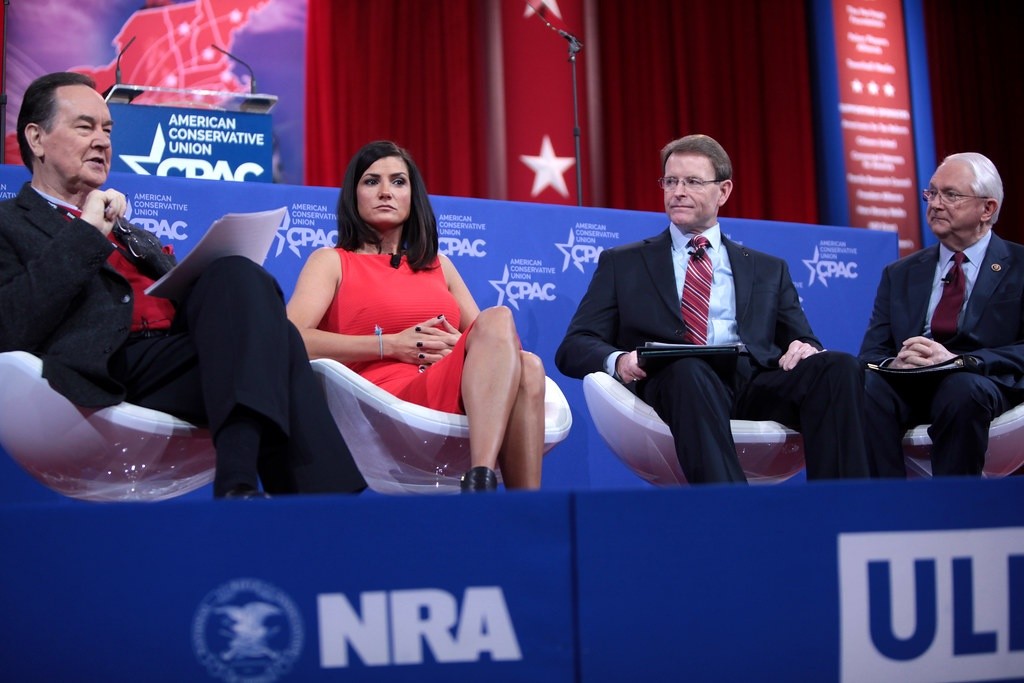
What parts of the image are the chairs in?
[309,358,571,493]
[903,402,1024,478]
[584,371,809,484]
[0,346,217,504]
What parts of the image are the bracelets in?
[375,325,385,361]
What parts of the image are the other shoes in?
[460,466,497,494]
[222,489,271,501]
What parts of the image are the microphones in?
[390,254,401,269]
[210,43,257,94]
[694,248,705,259]
[116,36,136,84]
[945,273,953,281]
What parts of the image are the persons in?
[555,136,861,485]
[856,150,1024,475]
[287,140,544,492]
[0,73,373,498]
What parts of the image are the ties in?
[931,252,966,344]
[680,234,713,346]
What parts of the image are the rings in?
[633,378,637,382]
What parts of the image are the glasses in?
[657,176,736,192]
[117,193,142,258]
[922,189,990,204]
